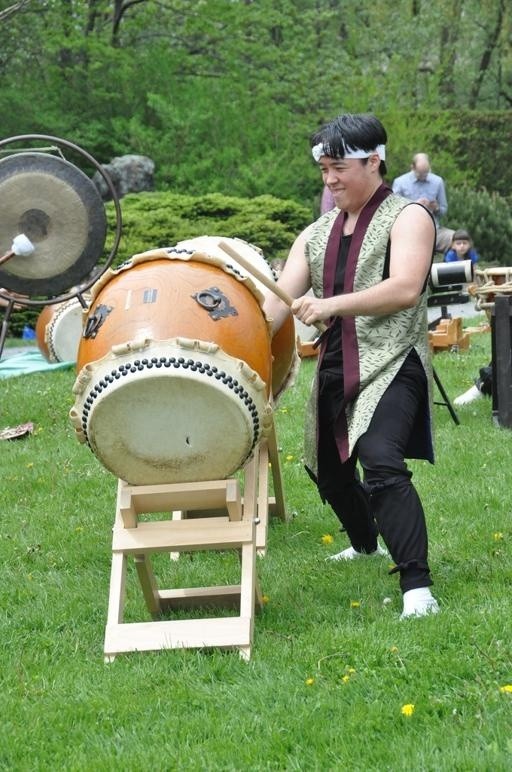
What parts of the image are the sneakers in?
[398,599,441,622]
[453,383,484,405]
[323,543,389,561]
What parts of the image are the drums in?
[175,235,301,408]
[36,294,95,365]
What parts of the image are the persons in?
[454,358,492,405]
[320,184,336,215]
[392,153,456,252]
[264,113,438,621]
[445,228,479,265]
[0,287,28,310]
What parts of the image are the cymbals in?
[0,152,108,297]
[69,247,275,485]
